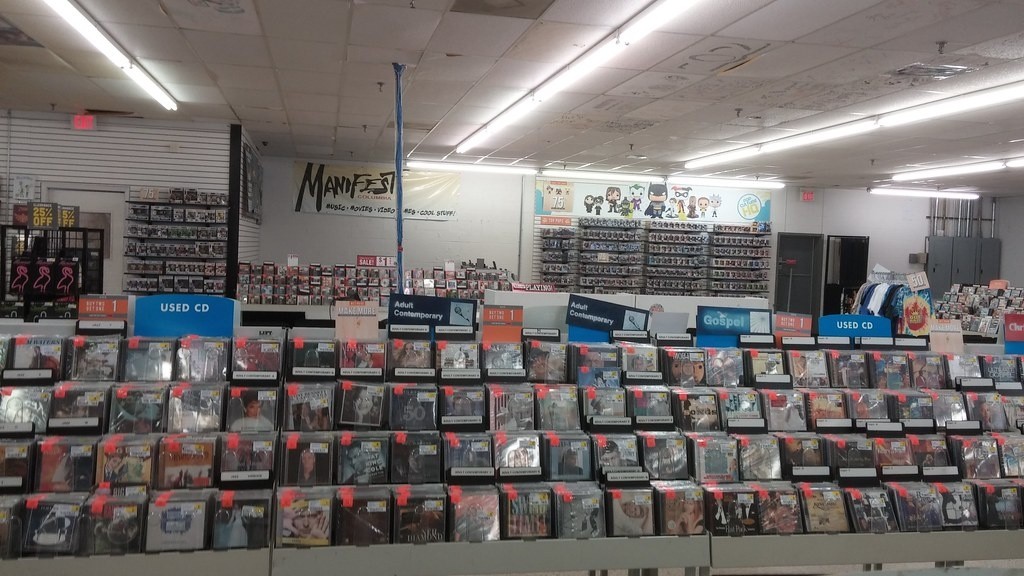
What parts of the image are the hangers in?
[864,272,909,285]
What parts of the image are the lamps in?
[405,159,787,189]
[456,0,703,154]
[866,188,982,200]
[44,0,178,112]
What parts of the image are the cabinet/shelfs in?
[122,200,229,295]
[644,228,703,291]
[576,226,644,288]
[539,236,576,287]
[707,232,772,294]
[928,236,1002,299]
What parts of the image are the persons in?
[4,336,1024,554]
